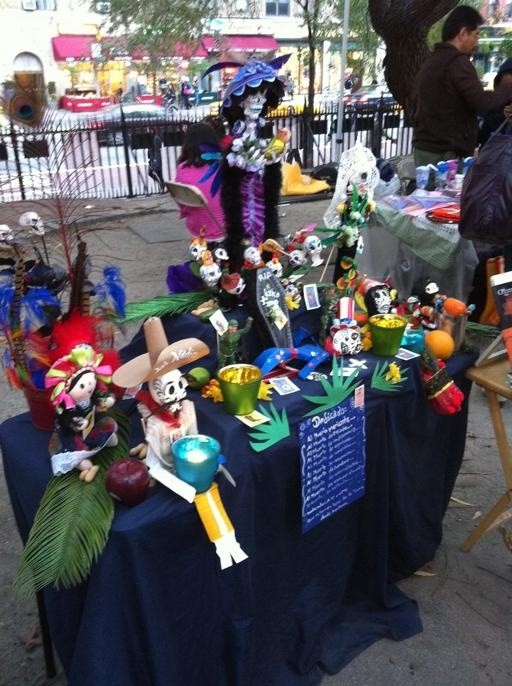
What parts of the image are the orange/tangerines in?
[187,367,210,388]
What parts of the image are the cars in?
[335,84,398,114]
[78,101,325,147]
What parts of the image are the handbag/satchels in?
[458,116,511,244]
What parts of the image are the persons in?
[462,56,512,324]
[286,72,294,100]
[161,75,201,117]
[370,75,378,85]
[344,78,353,89]
[404,4,488,166]
[147,126,165,194]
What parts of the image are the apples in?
[105,460,149,503]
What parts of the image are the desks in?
[1,302,479,684]
[359,173,492,321]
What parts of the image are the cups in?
[369,313,407,357]
[216,363,263,415]
[434,303,467,353]
[173,435,222,493]
